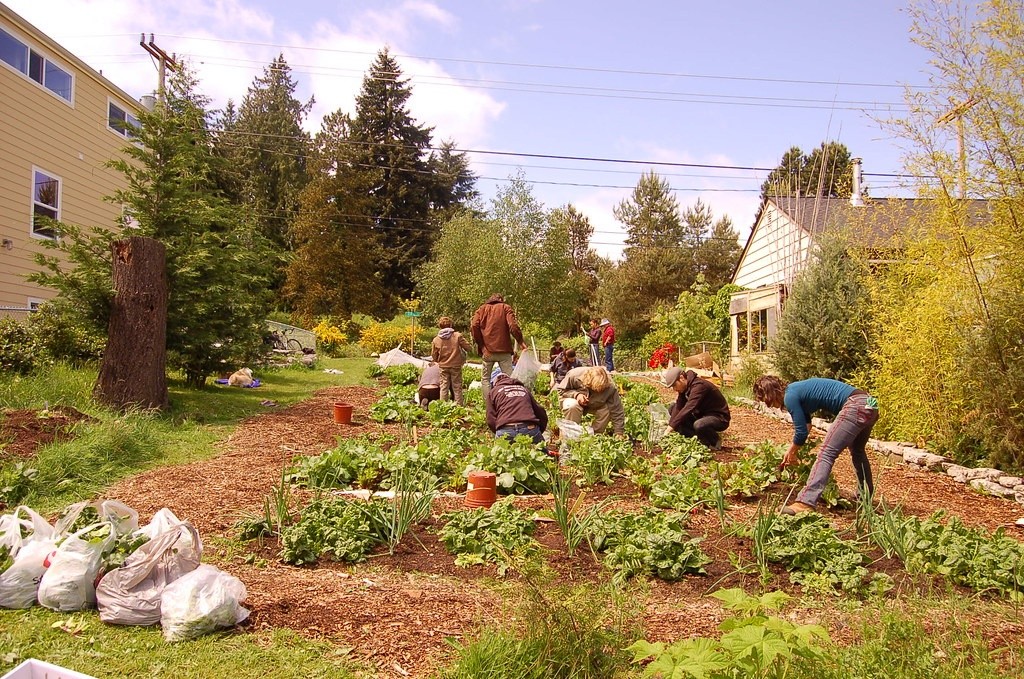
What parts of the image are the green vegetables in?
[0,506,152,574]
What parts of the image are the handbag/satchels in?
[509,349,541,387]
[0,500,251,642]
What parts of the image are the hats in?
[666,367,683,388]
[599,318,610,326]
[493,373,509,387]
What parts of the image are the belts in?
[502,422,531,429]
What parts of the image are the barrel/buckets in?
[334,402,353,424]
[464,470,496,509]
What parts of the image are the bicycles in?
[273,328,302,351]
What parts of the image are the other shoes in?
[420,398,428,411]
[778,502,815,516]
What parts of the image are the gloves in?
[865,397,879,411]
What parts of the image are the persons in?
[472,294,524,399]
[663,368,731,451]
[550,348,582,387]
[558,366,625,442]
[580,319,602,366]
[490,352,518,388]
[428,316,476,408]
[417,364,453,411]
[486,372,548,453]
[549,342,564,364]
[599,318,615,373]
[752,375,879,516]
